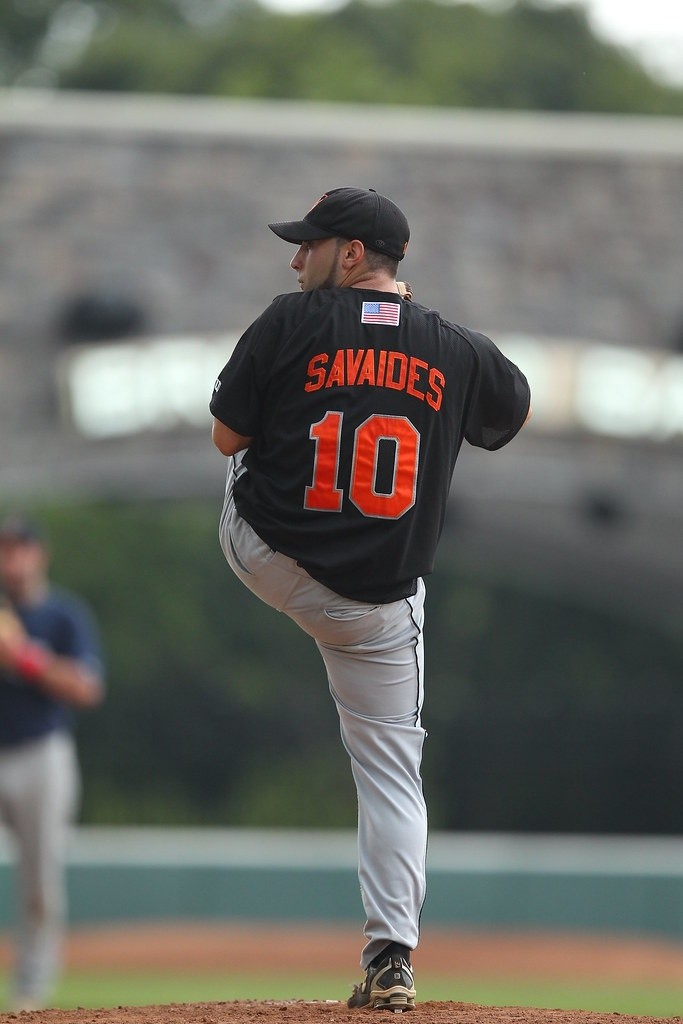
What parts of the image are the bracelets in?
[17,641,49,681]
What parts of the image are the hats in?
[268,188,409,261]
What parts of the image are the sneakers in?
[343,953,417,1012]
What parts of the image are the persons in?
[206,186,532,1009]
[0,519,106,1004]
